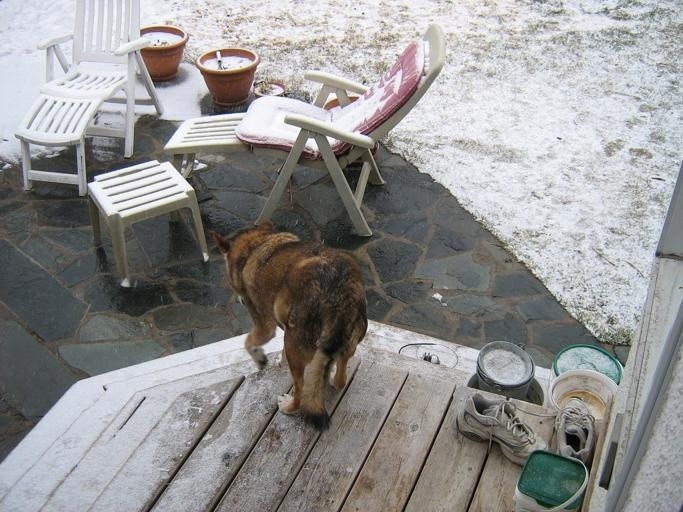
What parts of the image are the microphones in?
[82,160,212,290]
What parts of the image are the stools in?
[82,160,212,290]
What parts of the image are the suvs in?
[550,344,623,383]
[511,449,591,511]
[475,341,536,398]
[550,370,619,432]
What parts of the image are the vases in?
[140,26,190,81]
[198,46,260,106]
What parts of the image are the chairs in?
[17,1,162,197]
[554,397,594,465]
[163,22,445,237]
[455,392,556,467]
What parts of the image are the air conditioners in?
[207,219,369,432]
